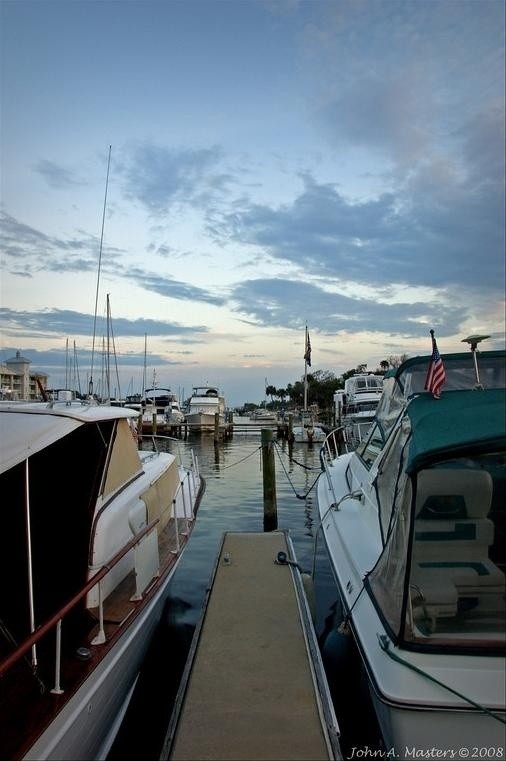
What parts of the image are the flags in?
[424,338,448,400]
[304,333,312,367]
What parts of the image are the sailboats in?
[0,292,506,440]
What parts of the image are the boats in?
[311,332,505,760]
[2,400,207,760]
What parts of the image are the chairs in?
[416,468,506,617]
